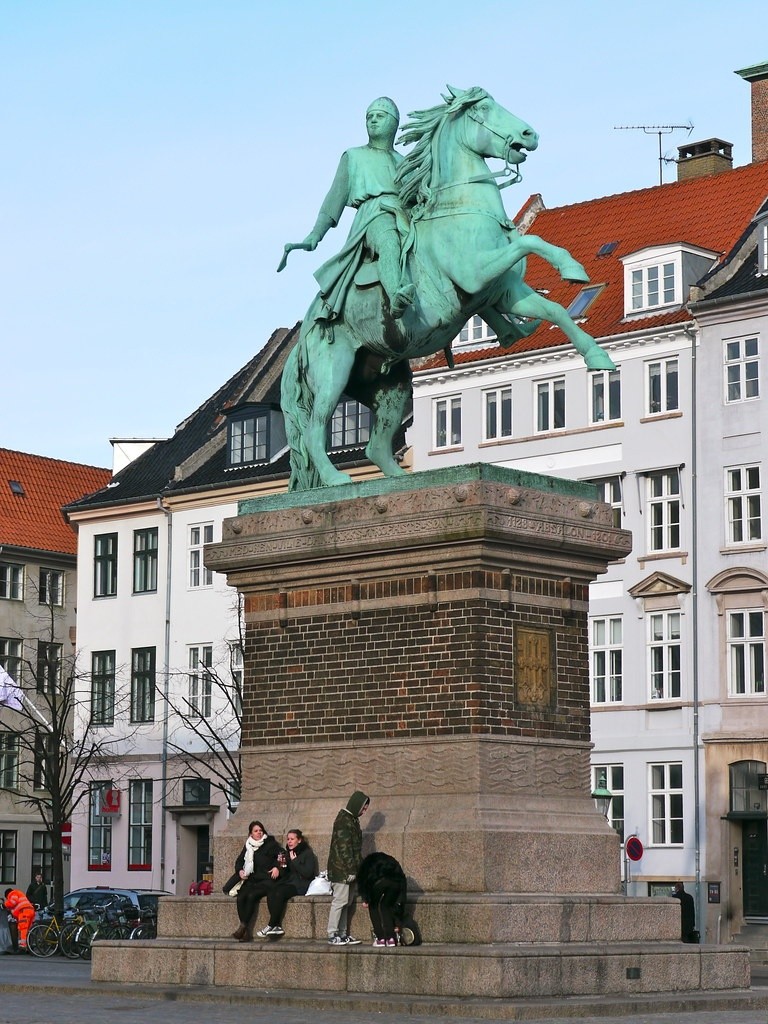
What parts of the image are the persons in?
[302,96,426,319]
[232,820,287,942]
[671,882,696,942]
[1,888,35,955]
[25,873,48,909]
[257,828,320,938]
[356,851,408,948]
[326,790,370,946]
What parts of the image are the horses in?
[278,82,619,494]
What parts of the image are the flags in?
[0,666,24,711]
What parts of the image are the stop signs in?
[61,823,72,846]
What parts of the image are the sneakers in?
[373,938,395,947]
[343,936,362,945]
[257,925,285,937]
[233,924,252,941]
[328,936,347,945]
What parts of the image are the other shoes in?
[17,948,30,954]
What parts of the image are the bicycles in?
[25,903,157,960]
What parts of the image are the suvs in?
[33,886,176,921]
